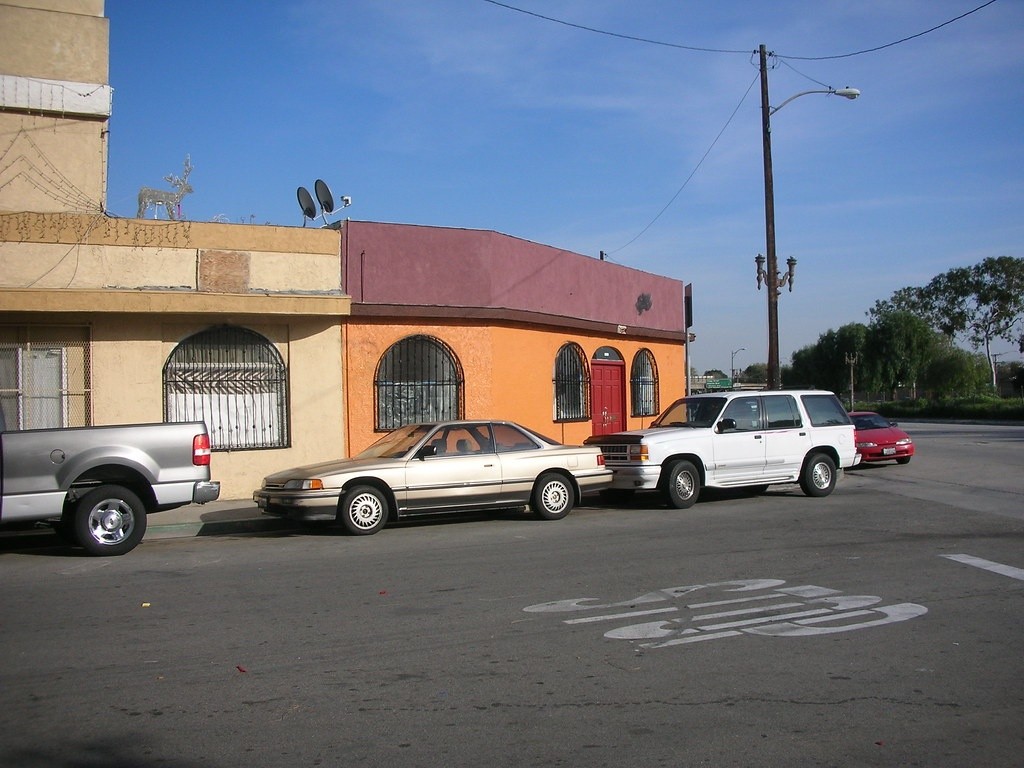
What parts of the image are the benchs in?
[496,443,533,451]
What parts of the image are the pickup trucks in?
[0,405,221,556]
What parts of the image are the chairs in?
[431,439,447,456]
[456,439,472,455]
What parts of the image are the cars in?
[849,412,915,464]
[252,419,615,536]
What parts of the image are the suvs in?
[582,390,863,509]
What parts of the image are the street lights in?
[732,347,747,391]
[838,351,858,412]
[755,85,861,392]
